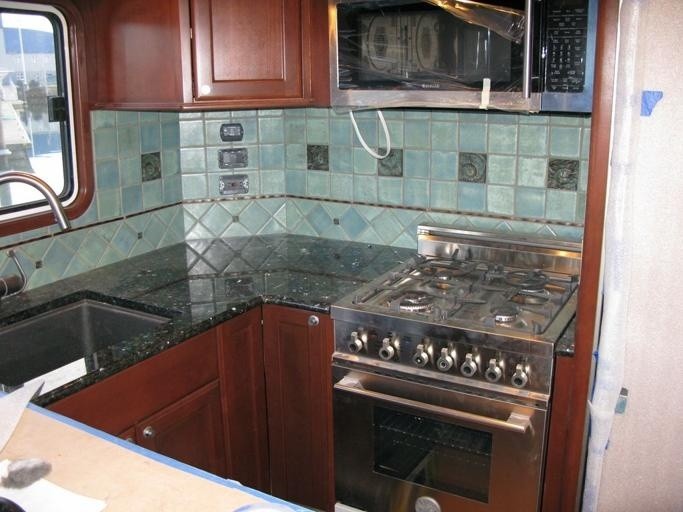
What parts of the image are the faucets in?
[0,172,71,234]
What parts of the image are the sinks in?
[0,290,182,387]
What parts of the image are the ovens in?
[327,362,551,512]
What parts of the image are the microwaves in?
[327,0,598,116]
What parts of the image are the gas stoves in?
[322,252,579,408]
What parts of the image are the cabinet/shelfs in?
[83,0,332,112]
[43,304,334,512]
